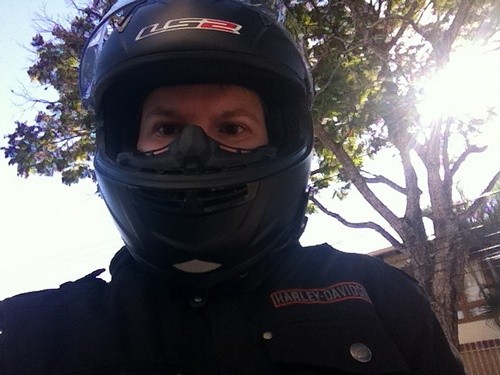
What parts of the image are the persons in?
[0,1,468,375]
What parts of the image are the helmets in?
[81,1,316,287]
